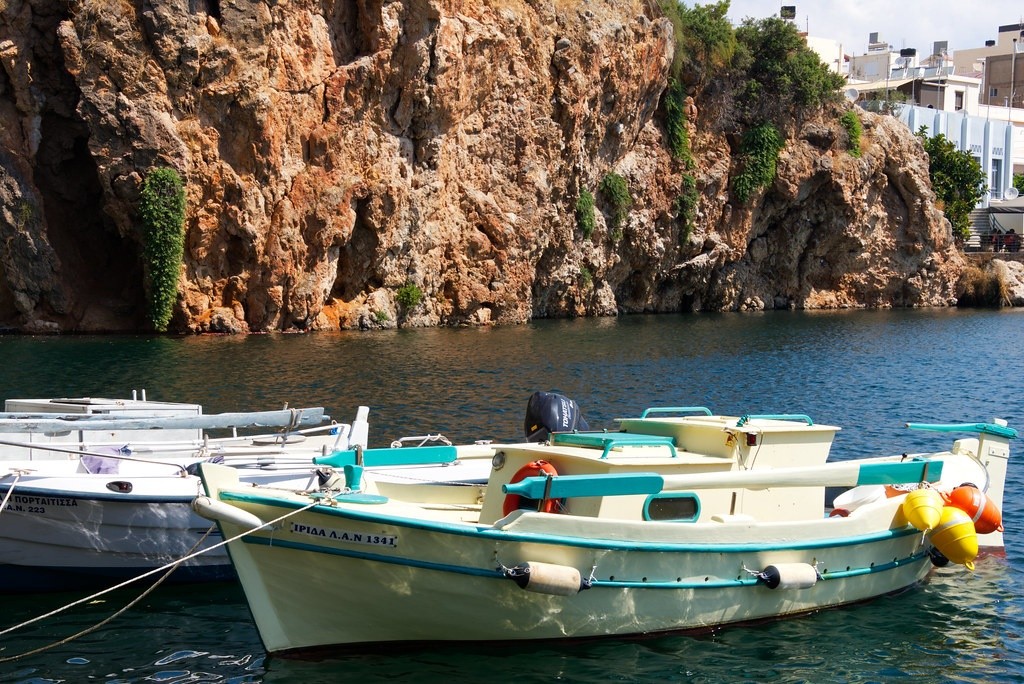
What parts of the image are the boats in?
[0,385,590,571]
[187,408,1021,657]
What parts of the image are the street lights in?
[1008,37,1019,123]
[937,53,943,110]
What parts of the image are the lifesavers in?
[503,460,561,516]
